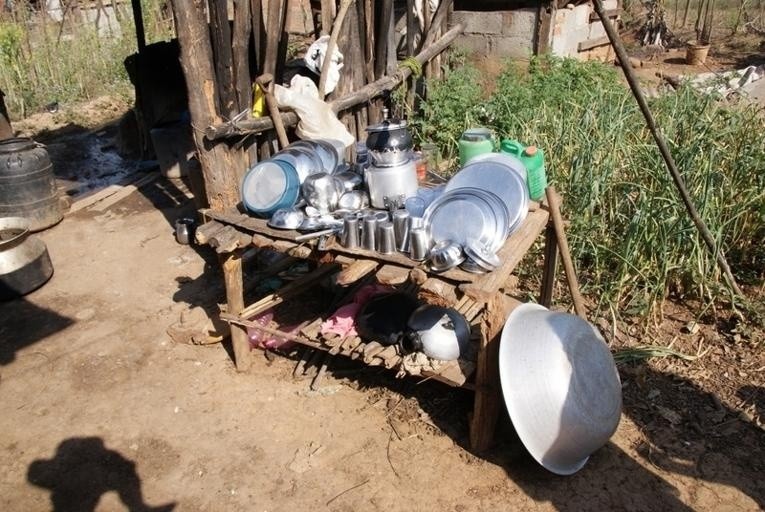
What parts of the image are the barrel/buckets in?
[497,138,550,204]
[1,136,65,232]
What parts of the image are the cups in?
[340,216,359,248]
[360,216,378,250]
[374,212,391,223]
[409,228,430,261]
[398,216,424,254]
[376,222,397,255]
[392,209,409,246]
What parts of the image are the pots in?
[355,278,421,347]
[399,304,473,361]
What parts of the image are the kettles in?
[364,118,419,209]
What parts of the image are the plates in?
[449,187,510,255]
[443,162,530,240]
[461,152,528,187]
[421,192,498,255]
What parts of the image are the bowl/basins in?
[499,302,622,475]
[268,146,324,209]
[286,139,339,178]
[242,159,300,219]
[337,190,371,213]
[321,139,351,175]
[430,239,467,272]
[267,208,305,229]
